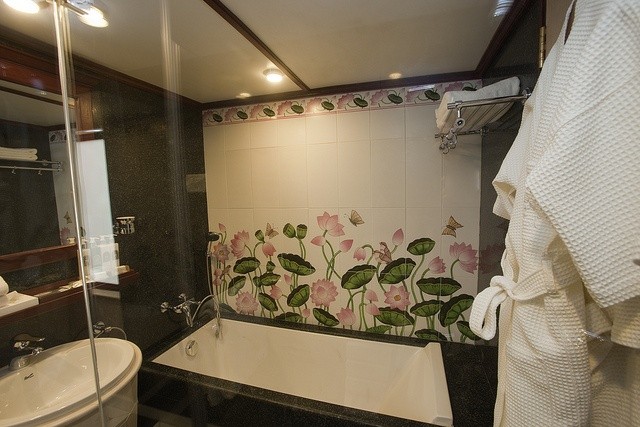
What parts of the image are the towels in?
[0,278,9,307]
[0,146,39,161]
[434,76,520,134]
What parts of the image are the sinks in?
[0,338,142,426]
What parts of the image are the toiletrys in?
[76,234,120,278]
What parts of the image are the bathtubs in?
[152,317,453,427]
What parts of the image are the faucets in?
[169,295,200,327]
[11,334,45,368]
